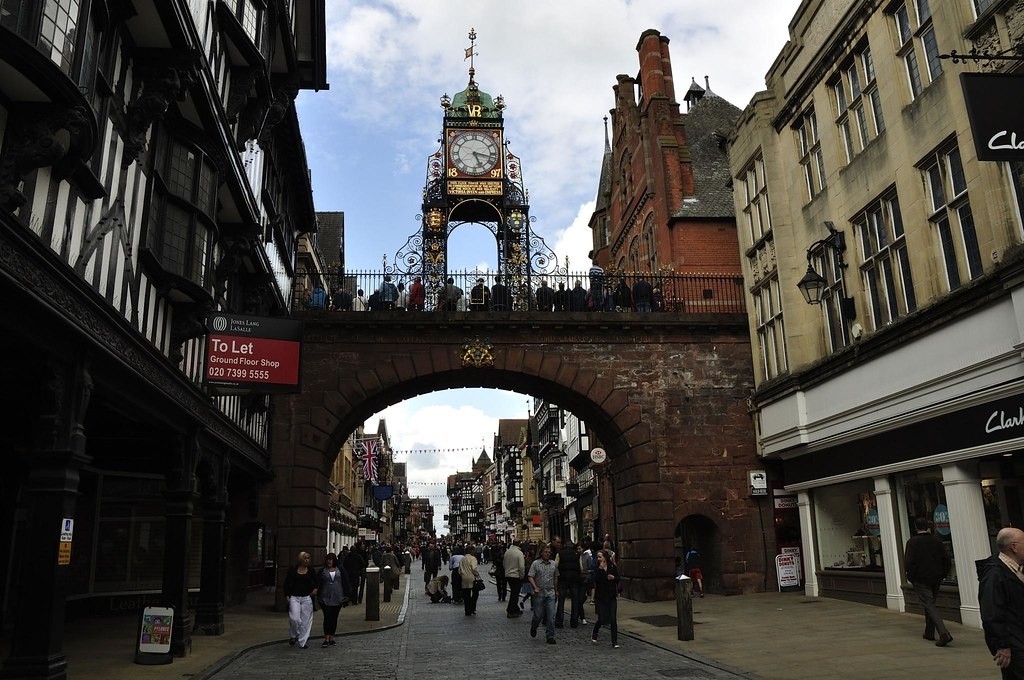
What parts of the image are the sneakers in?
[614,644,620,649]
[579,618,588,625]
[322,640,330,647]
[590,636,598,643]
[329,640,337,646]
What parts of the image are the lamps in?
[797,240,848,305]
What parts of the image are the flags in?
[362,439,381,481]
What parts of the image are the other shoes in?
[352,602,358,605]
[507,610,522,618]
[691,591,696,598]
[289,637,298,647]
[589,600,595,605]
[700,592,704,598]
[298,642,309,649]
[465,611,476,616]
[520,602,524,609]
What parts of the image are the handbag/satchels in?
[472,574,485,592]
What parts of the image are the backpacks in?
[688,550,699,568]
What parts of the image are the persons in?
[975,527,1024,680]
[904,519,952,647]
[686,546,704,597]
[284,530,624,649]
[309,272,664,312]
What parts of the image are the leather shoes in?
[546,638,556,644]
[530,622,539,637]
[935,632,954,647]
[923,633,935,641]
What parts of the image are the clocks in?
[450,132,499,177]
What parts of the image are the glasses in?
[596,555,603,559]
